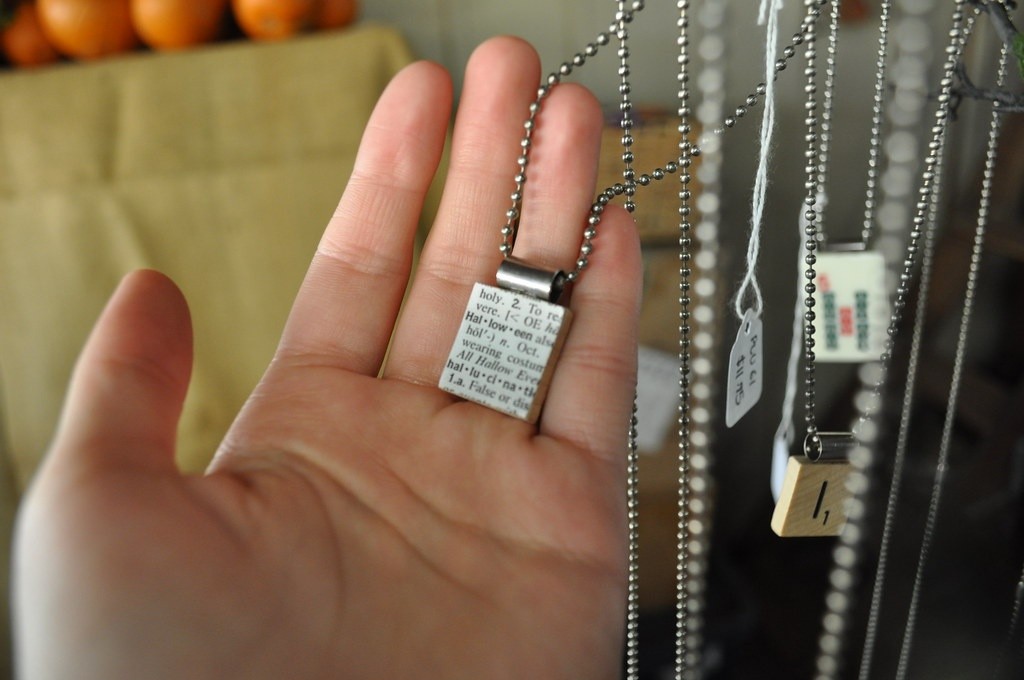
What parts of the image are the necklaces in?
[435,0,1013,680]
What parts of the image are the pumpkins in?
[0,0,357,71]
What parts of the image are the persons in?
[11,35,645,680]
